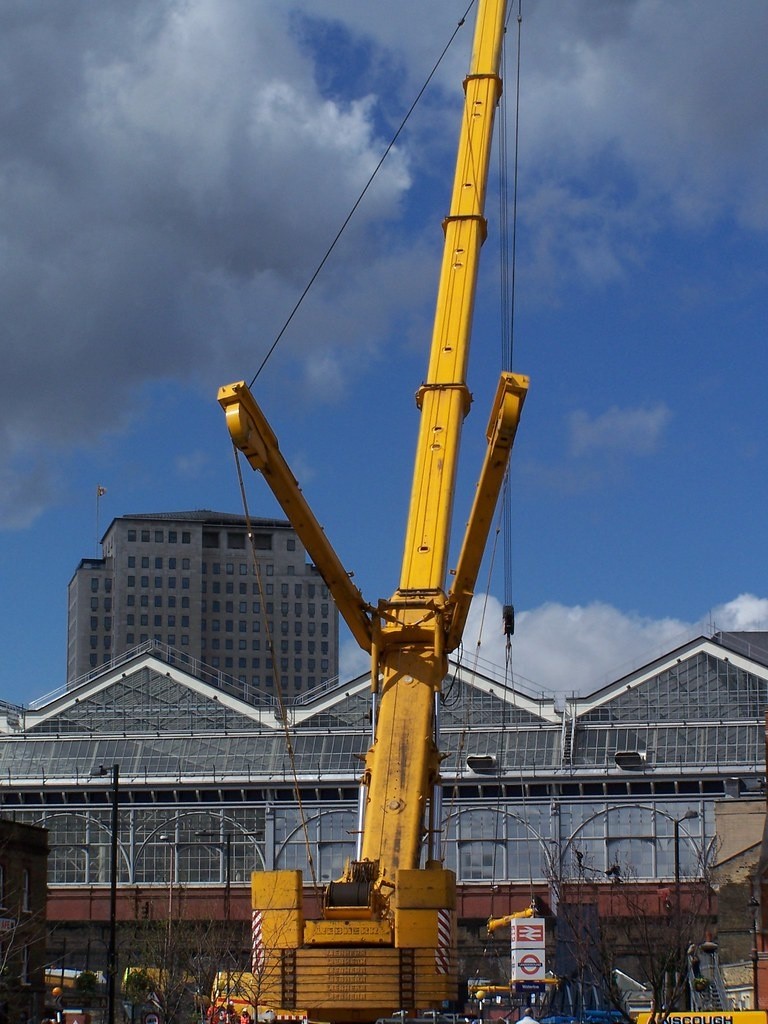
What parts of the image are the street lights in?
[670,813,703,1013]
[89,763,122,1024]
[194,830,263,1004]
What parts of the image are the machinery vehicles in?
[209,0,567,1024]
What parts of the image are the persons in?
[516,1008,540,1024]
[207,998,251,1024]
